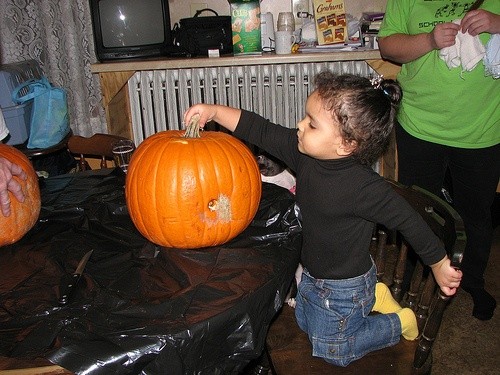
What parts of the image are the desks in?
[90,49,404,182]
[0,167,304,375]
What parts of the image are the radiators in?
[127,60,384,175]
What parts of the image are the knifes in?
[57,249,94,304]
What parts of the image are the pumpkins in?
[0,141,41,248]
[126,116,261,248]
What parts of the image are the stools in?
[0,126,73,178]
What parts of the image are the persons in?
[377,1,500,320]
[183,72,462,367]
[0,157,27,217]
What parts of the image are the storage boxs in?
[226,0,263,56]
[312,0,348,45]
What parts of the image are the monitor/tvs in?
[89,0,171,64]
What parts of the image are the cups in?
[274,31,296,55]
[112,140,137,176]
[277,12,295,32]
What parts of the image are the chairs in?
[266,178,466,375]
[68,133,136,171]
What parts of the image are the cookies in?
[232,7,260,45]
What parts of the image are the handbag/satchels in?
[181,9,234,58]
[13,76,71,149]
